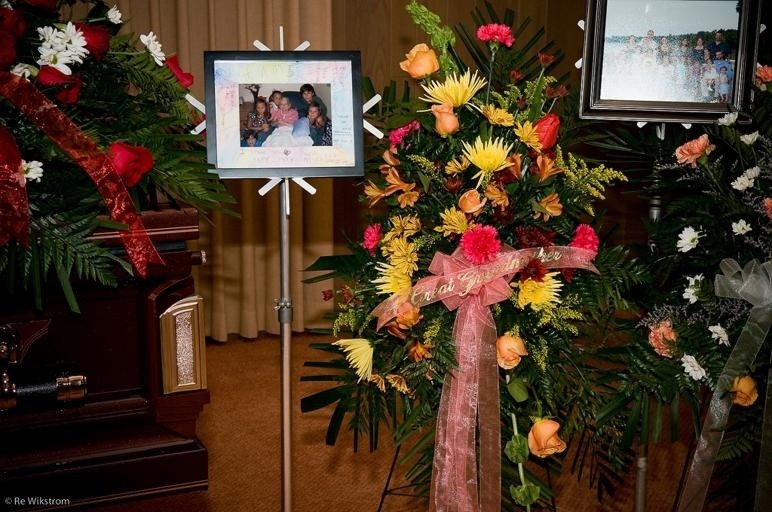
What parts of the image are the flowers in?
[0,0,243,315]
[296,1,771,510]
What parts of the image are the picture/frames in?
[204,50,365,179]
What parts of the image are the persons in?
[239,82,333,146]
[607,24,733,102]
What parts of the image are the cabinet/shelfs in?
[0,187,215,512]
